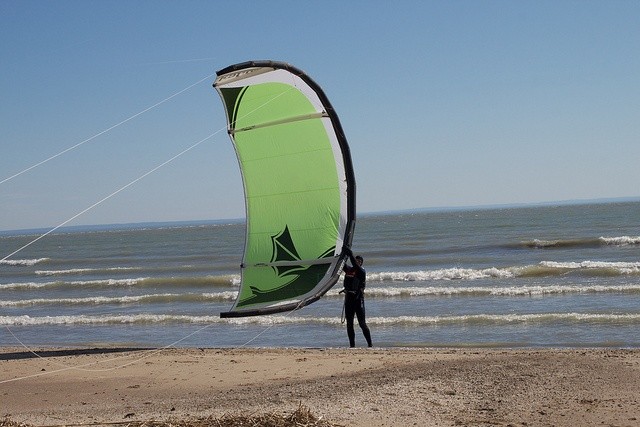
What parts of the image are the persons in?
[342,250,373,347]
